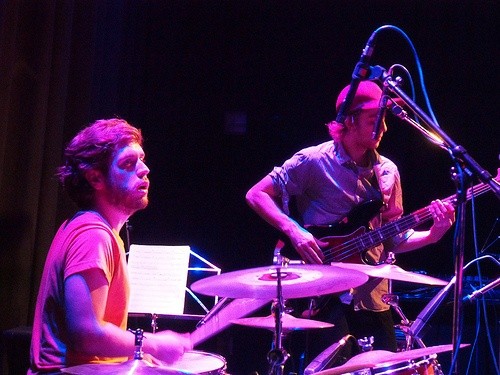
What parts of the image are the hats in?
[335,80,404,114]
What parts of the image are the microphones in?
[304,337,348,375]
[336,32,379,125]
[371,67,393,141]
[409,275,456,337]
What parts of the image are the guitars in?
[305,168,500,298]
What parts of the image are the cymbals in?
[331,261,449,286]
[229,313,335,336]
[190,264,368,299]
[310,343,471,375]
[60,359,201,375]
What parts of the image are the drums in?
[173,350,227,375]
[341,352,439,375]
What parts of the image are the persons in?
[24,119,191,375]
[243,82,455,374]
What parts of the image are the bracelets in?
[133,328,143,360]
